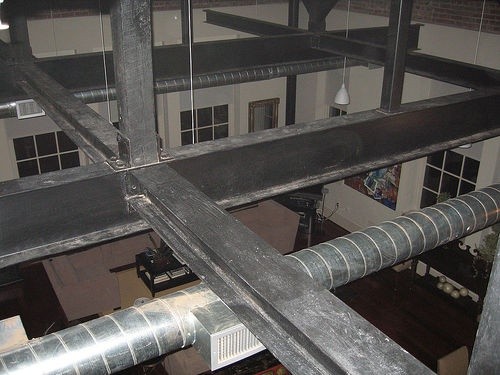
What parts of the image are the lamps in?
[334,0,351,105]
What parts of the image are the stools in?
[418,273,473,334]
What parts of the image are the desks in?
[135,248,200,298]
[410,239,493,315]
